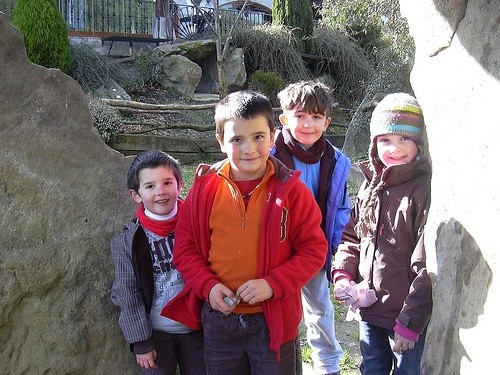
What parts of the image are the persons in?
[110,149,203,375]
[269,81,352,375]
[161,90,328,375]
[331,93,432,375]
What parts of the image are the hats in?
[369,92,427,158]
